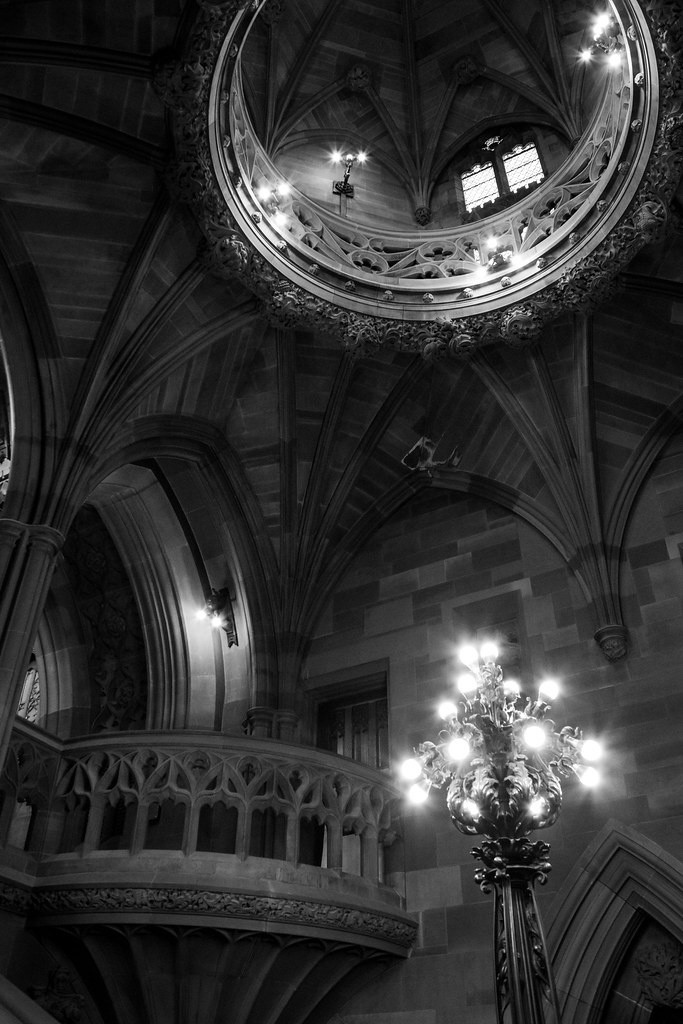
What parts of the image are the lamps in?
[330,150,369,198]
[193,586,239,647]
[398,630,608,1024]
[580,12,622,62]
